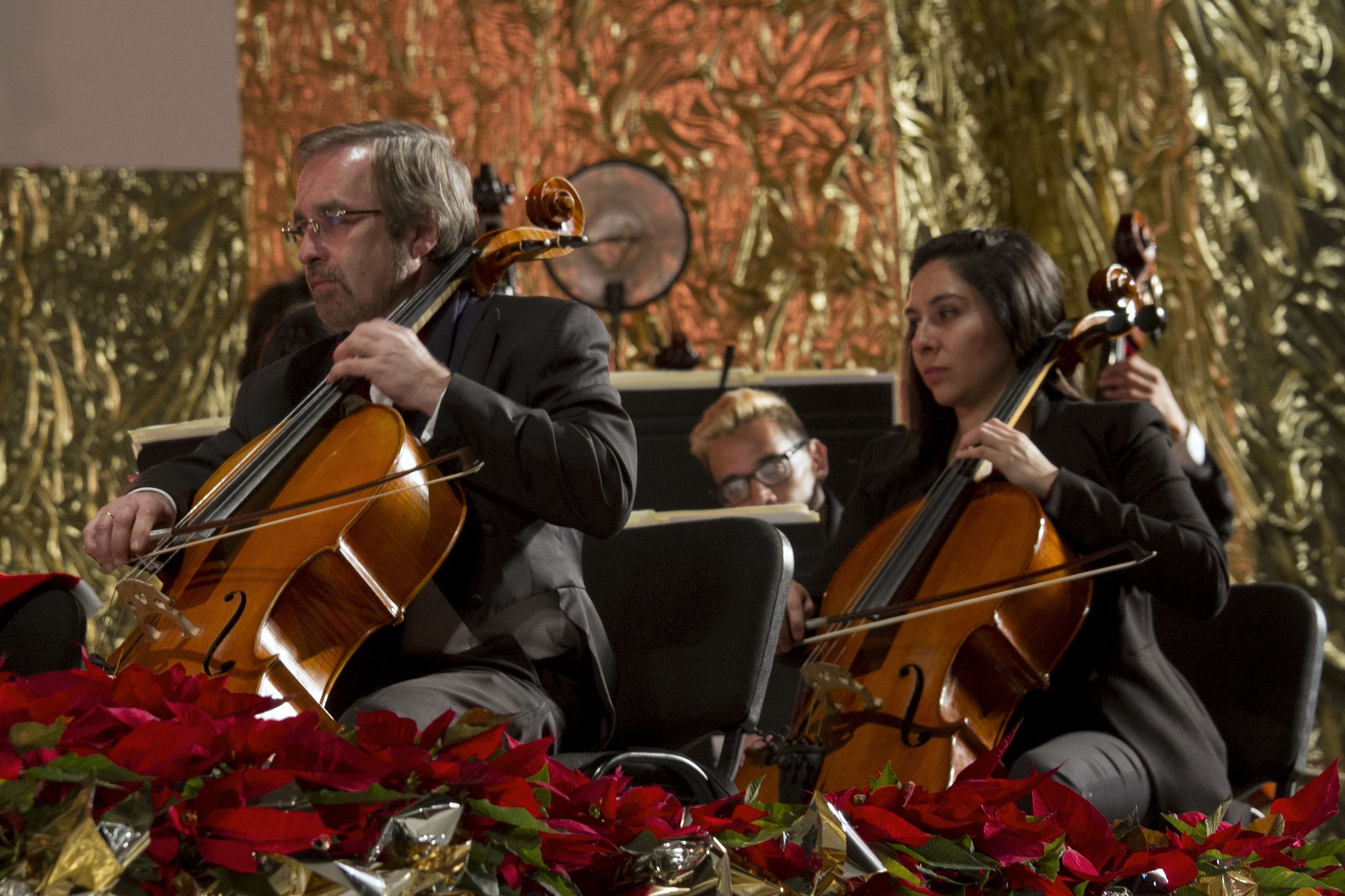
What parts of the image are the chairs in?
[577,515,795,798]
[1214,579,1332,802]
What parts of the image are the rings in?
[100,511,114,519]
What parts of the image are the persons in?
[770,223,1237,828]
[77,112,642,761]
[1102,354,1234,545]
[686,386,861,596]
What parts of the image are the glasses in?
[280,209,384,243]
[714,439,809,505]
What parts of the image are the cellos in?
[727,204,1174,822]
[93,174,589,734]
